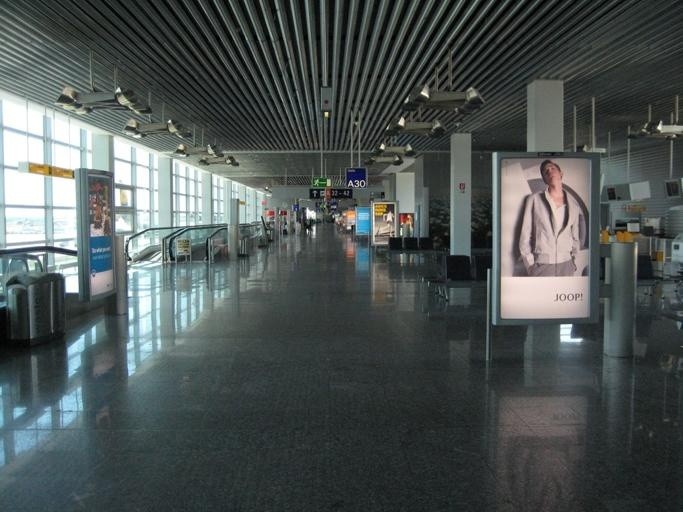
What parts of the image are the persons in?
[519,160,579,277]
[383,211,394,237]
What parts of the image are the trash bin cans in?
[238,239,248,257]
[6,270,65,348]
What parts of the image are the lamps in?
[362,43,485,166]
[577,94,683,154]
[54,50,238,169]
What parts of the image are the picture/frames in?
[356,207,370,236]
[491,151,599,326]
[370,200,399,246]
